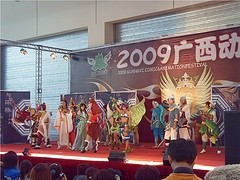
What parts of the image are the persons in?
[26,100,140,153]
[162,138,203,180]
[150,96,191,154]
[200,101,222,154]
[0,151,118,180]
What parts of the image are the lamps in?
[20,48,27,55]
[63,54,69,61]
[50,52,57,59]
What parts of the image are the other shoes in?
[46,145,51,148]
[162,151,165,154]
[57,147,60,150]
[200,149,206,153]
[81,150,84,152]
[33,146,40,149]
[123,149,131,152]
[152,146,159,148]
[217,150,221,154]
